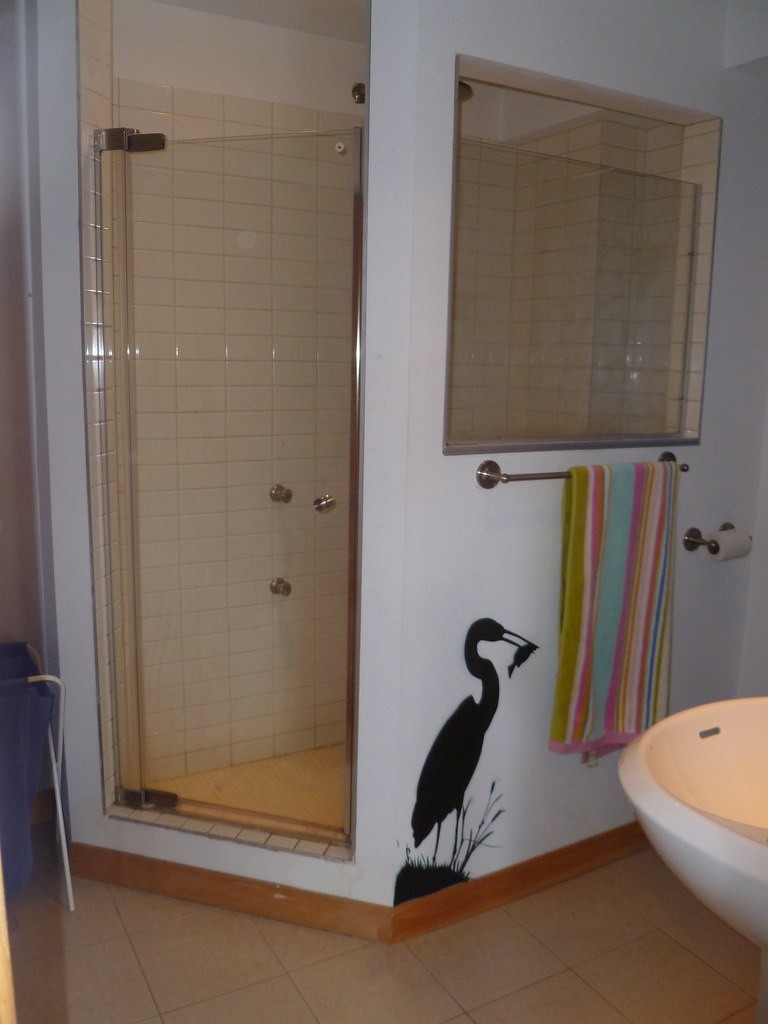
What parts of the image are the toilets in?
[617,696,768,1024]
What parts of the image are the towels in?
[547,460,680,768]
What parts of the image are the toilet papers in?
[703,527,753,563]
